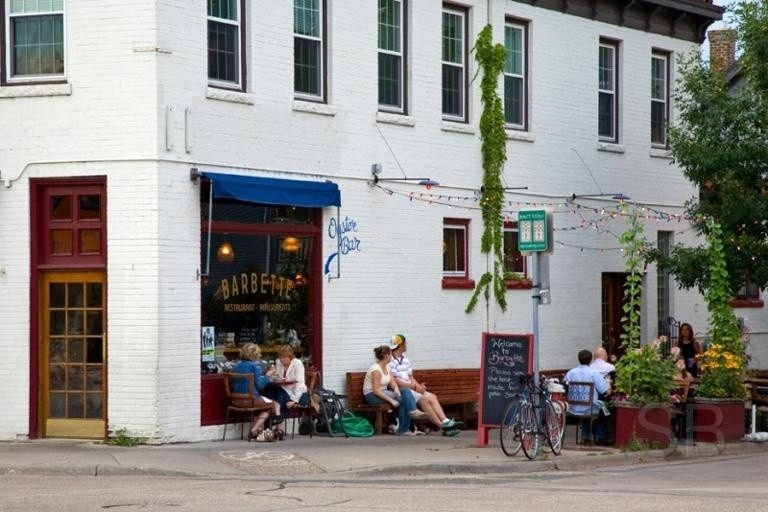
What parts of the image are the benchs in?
[538,369,572,386]
[346,366,483,438]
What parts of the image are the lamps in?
[219,244,236,261]
[281,234,301,252]
[372,173,438,193]
[572,191,628,205]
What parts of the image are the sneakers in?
[443,428,461,436]
[407,409,429,420]
[400,429,416,436]
[441,417,465,431]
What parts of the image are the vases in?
[688,396,747,442]
[615,405,671,449]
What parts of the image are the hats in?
[388,334,406,350]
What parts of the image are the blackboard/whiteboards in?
[482,335,529,425]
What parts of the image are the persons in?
[590,347,615,380]
[563,350,614,446]
[261,342,310,429]
[672,322,702,378]
[229,342,284,441]
[672,355,697,383]
[387,334,465,437]
[362,346,430,437]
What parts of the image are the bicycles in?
[501,376,566,456]
[517,378,563,458]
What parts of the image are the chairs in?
[564,380,604,443]
[219,373,274,442]
[282,367,323,441]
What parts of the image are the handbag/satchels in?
[256,428,280,442]
[329,411,374,437]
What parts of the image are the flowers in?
[615,335,686,403]
[698,345,742,395]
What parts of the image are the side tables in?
[265,372,298,430]
